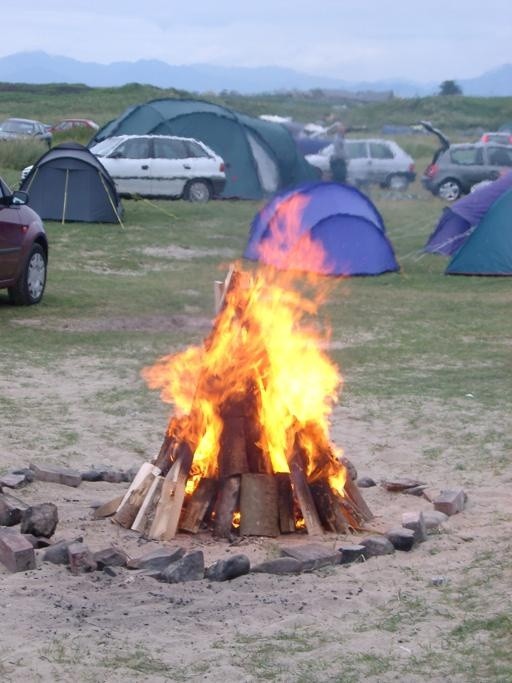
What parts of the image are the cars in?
[1,115,103,148]
[1,177,50,305]
[303,138,415,195]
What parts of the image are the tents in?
[85,97,321,198]
[17,141,121,223]
[242,180,400,275]
[424,171,512,275]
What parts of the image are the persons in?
[327,122,351,184]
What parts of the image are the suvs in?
[21,133,228,199]
[418,119,511,200]
